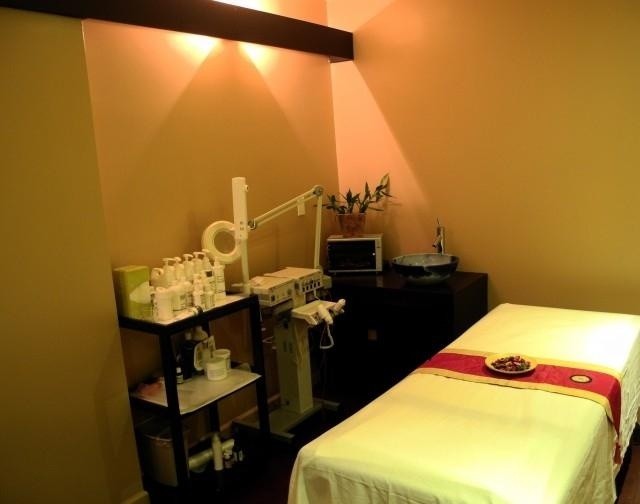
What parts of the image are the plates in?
[483,351,539,376]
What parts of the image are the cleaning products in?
[182,325,207,357]
[151,248,225,321]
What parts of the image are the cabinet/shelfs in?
[116,288,272,504]
[314,266,489,401]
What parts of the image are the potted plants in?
[311,172,395,238]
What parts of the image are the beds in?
[287,300,639,503]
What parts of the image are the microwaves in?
[326,233,384,273]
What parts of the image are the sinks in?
[392,253,460,283]
[432,217,444,254]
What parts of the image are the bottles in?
[176,326,230,384]
